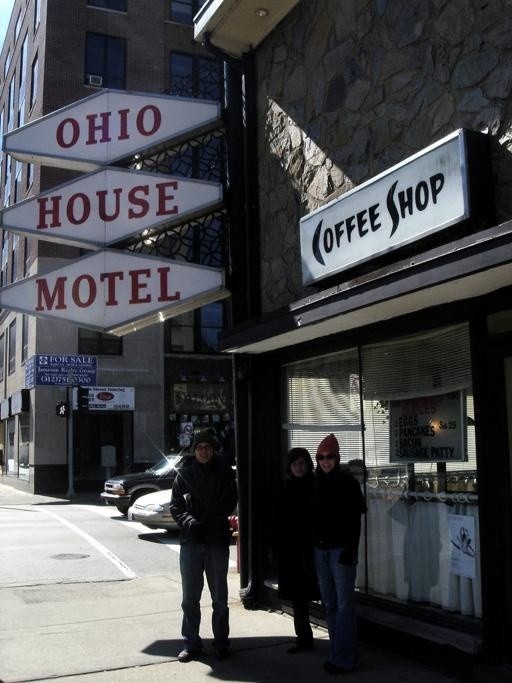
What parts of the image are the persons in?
[305,433,369,674]
[259,448,324,653]
[169,431,238,663]
[403,475,440,602]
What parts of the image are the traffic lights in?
[56,401,69,418]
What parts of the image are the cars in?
[99,454,238,529]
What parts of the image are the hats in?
[315,433,340,462]
[192,426,216,450]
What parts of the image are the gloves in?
[180,514,208,539]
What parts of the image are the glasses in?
[317,453,335,461]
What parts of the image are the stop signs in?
[181,422,194,435]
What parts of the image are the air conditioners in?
[88,74,103,85]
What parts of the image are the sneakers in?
[175,639,363,676]
